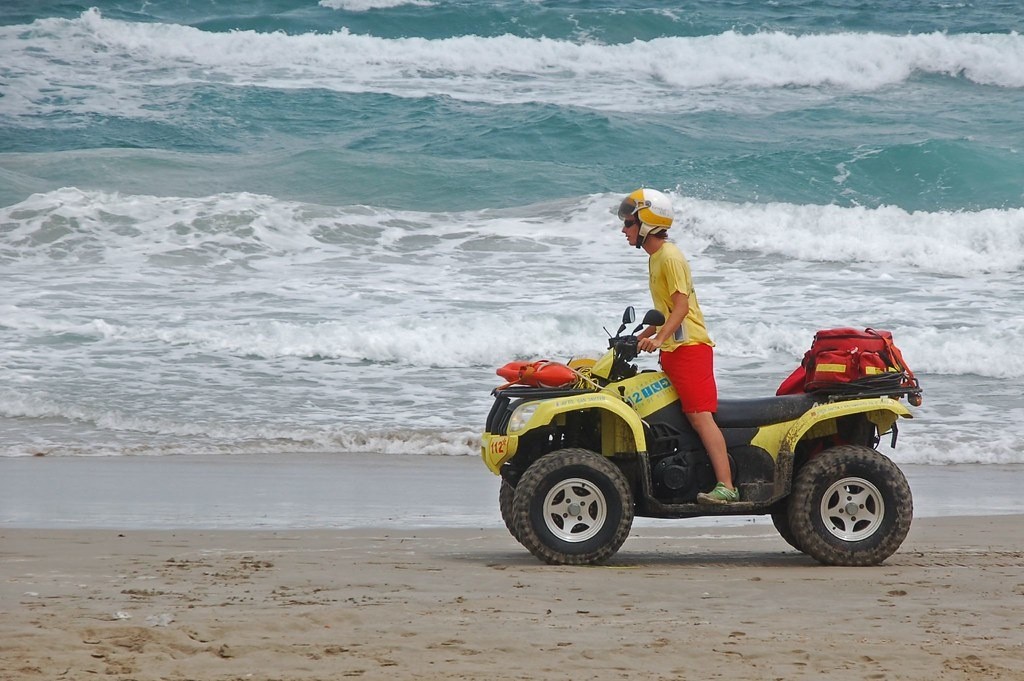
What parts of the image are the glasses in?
[623,219,639,228]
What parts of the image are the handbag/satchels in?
[803,327,907,394]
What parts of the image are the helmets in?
[624,188,674,228]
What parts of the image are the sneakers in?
[697,482,740,505]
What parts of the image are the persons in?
[609,187,740,505]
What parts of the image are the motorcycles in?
[478,306,925,570]
